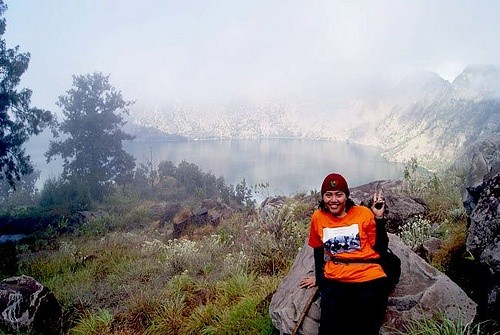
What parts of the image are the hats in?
[321,172,349,195]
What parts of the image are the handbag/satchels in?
[331,251,401,291]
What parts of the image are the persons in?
[299,173,390,335]
